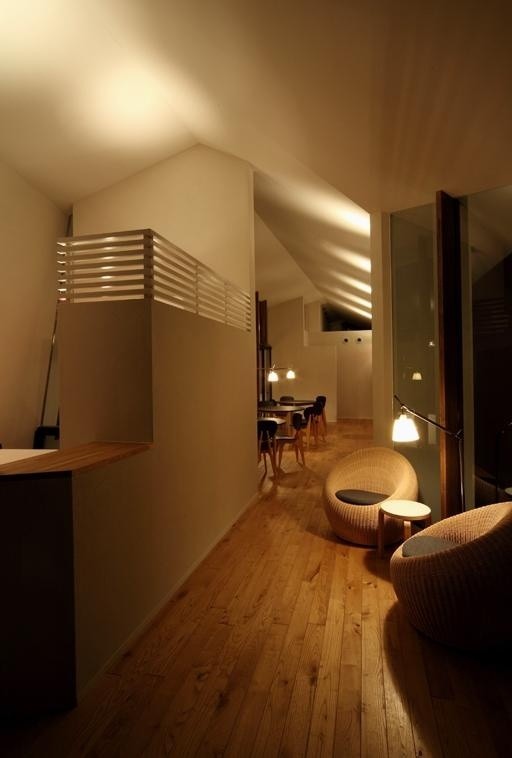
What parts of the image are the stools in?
[377,499,432,559]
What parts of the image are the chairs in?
[257,395,327,479]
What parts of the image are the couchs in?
[389,501,512,646]
[322,447,419,546]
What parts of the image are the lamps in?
[257,361,279,382]
[391,395,464,511]
[270,362,296,379]
